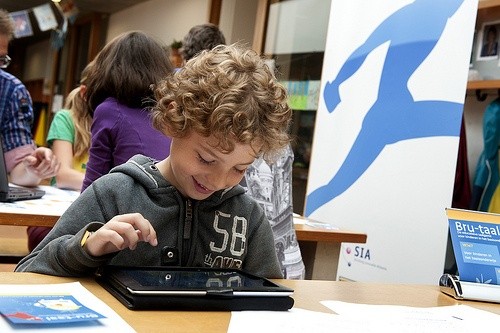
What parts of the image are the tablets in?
[100,265,294,311]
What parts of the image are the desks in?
[0,184,367,280]
[0,272,500,333]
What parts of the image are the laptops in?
[0,134,45,203]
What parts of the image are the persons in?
[46,23,305,280]
[13,44,282,279]
[0,8,61,187]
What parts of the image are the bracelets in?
[81,230,94,248]
